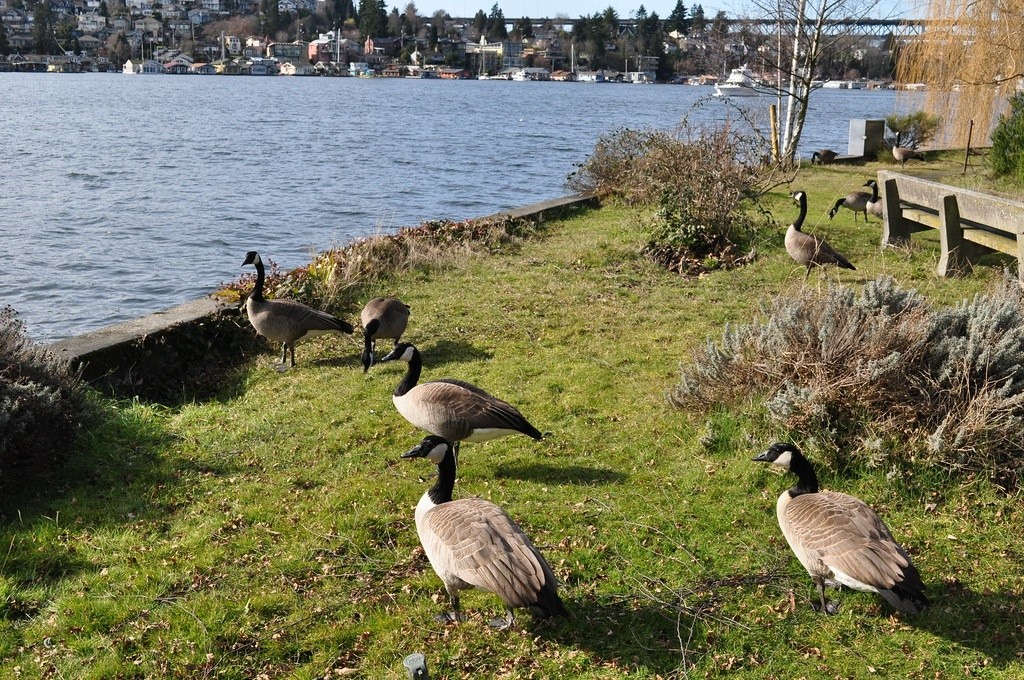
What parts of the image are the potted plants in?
[884,111,945,160]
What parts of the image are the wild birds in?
[783,190,856,285]
[862,179,883,220]
[361,291,411,375]
[400,436,567,638]
[827,191,872,222]
[377,340,543,473]
[749,439,927,622]
[242,247,354,372]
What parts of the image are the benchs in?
[876,169,1024,287]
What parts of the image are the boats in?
[714,62,760,97]
